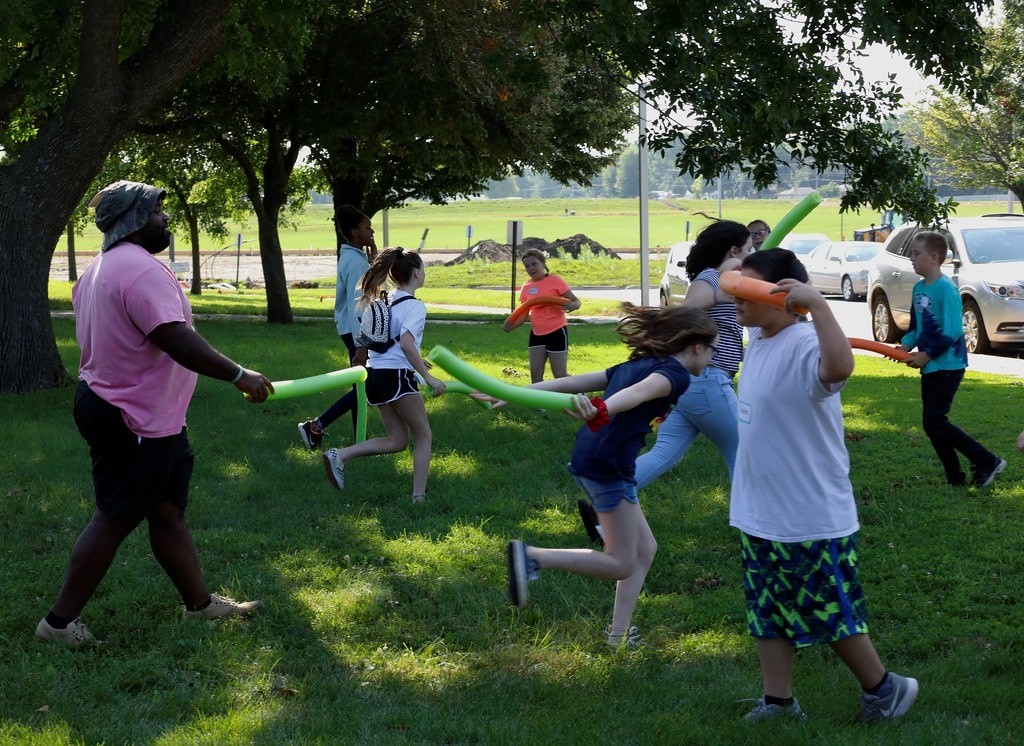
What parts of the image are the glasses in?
[751,228,767,240]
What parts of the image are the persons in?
[884,231,1007,487]
[580,221,752,550]
[469,302,717,653]
[35,180,275,650]
[731,218,918,727]
[503,250,588,413]
[322,248,446,505]
[298,205,379,451]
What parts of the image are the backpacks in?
[355,290,418,354]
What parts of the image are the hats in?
[90,180,167,252]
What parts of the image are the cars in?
[867,217,1024,355]
[659,244,693,308]
[749,235,832,265]
[800,241,884,302]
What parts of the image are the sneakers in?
[323,448,346,491]
[853,672,918,724]
[411,494,431,506]
[35,617,108,652]
[182,592,265,626]
[298,420,325,450]
[741,695,808,729]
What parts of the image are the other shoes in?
[508,539,540,610]
[969,454,1006,488]
[577,498,605,548]
[603,626,651,653]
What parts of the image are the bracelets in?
[585,395,610,434]
[229,364,243,384]
